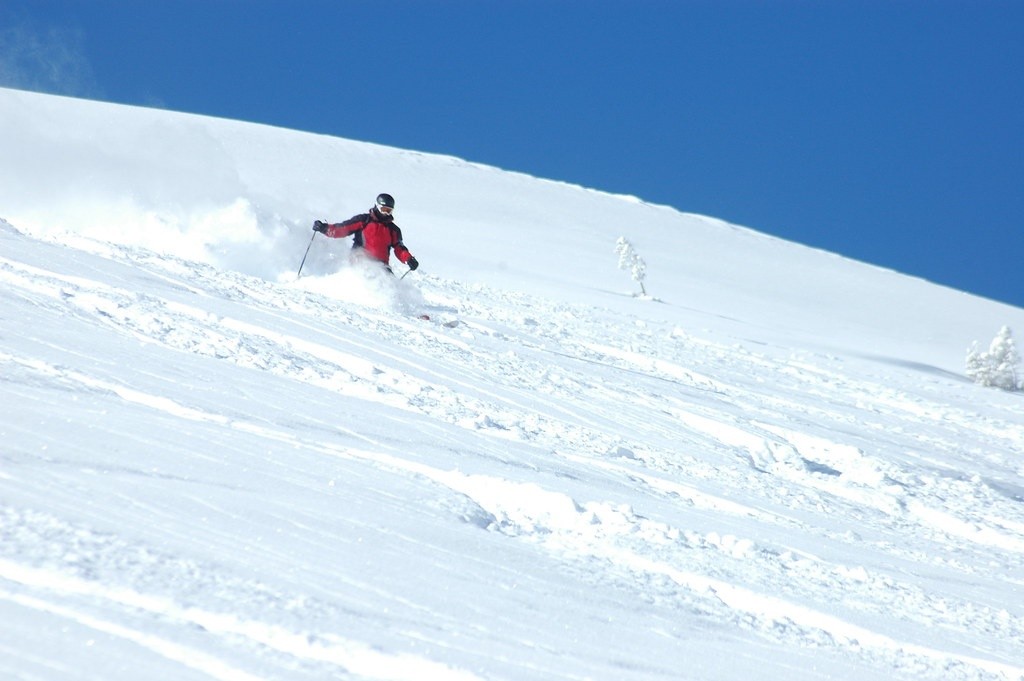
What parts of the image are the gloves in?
[408,256,418,270]
[312,220,328,234]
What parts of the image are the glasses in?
[375,201,393,215]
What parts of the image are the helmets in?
[375,193,395,210]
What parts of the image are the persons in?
[312,192,419,276]
